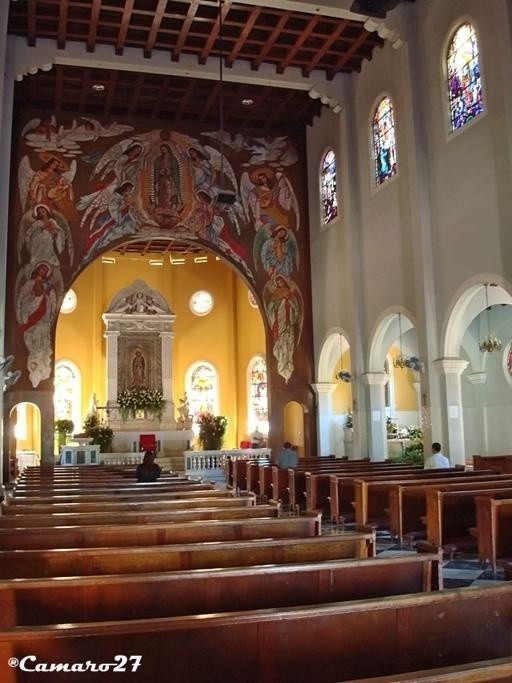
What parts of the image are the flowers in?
[196,409,227,448]
[117,388,167,422]
[388,419,423,463]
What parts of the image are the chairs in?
[138,433,159,456]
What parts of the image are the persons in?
[135,451,161,482]
[85,393,100,422]
[132,349,145,385]
[248,136,310,381]
[423,443,450,471]
[19,131,76,382]
[116,291,165,314]
[275,441,299,474]
[107,123,232,237]
[178,391,190,417]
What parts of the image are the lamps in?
[478,282,503,353]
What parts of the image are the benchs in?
[0,455,512,683]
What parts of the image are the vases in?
[202,439,221,450]
[122,407,156,421]
